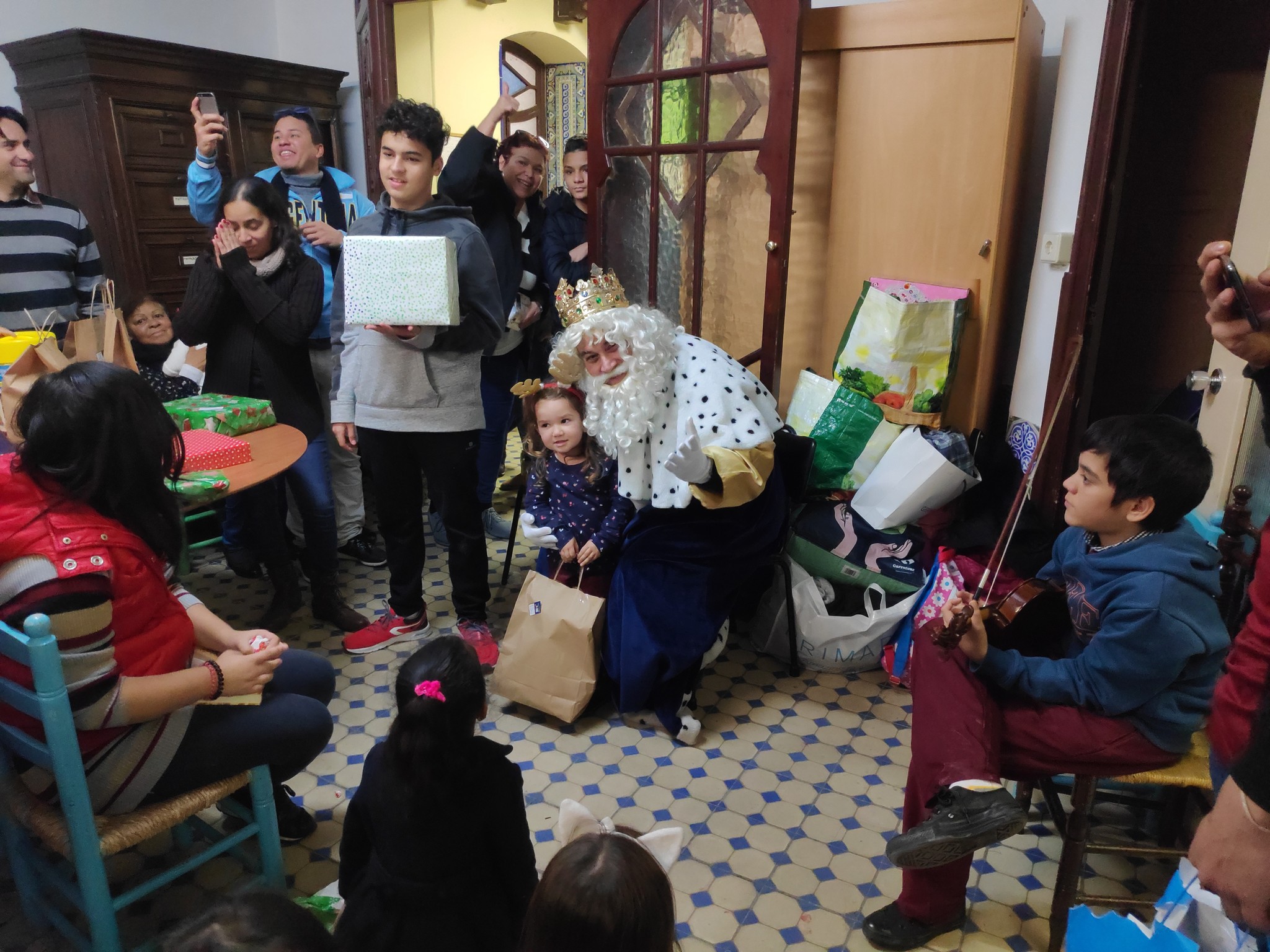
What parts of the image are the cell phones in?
[197,92,223,137]
[1218,249,1261,334]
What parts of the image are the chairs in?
[1016,484,1270,952]
[0,611,298,952]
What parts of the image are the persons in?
[1,81,1270,952]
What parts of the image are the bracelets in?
[205,661,225,700]
[197,661,218,700]
[1240,791,1269,834]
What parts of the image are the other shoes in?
[337,534,387,566]
[216,782,318,842]
[862,900,967,950]
[221,541,262,578]
[501,473,527,490]
[885,785,1028,870]
[499,463,506,476]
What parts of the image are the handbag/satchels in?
[784,279,985,674]
[63,278,140,374]
[490,554,606,722]
[1067,856,1270,952]
[1,309,72,443]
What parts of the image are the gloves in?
[663,416,715,484]
[520,513,560,550]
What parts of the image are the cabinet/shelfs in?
[679,0,1051,445]
[0,29,350,339]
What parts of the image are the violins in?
[927,578,1060,665]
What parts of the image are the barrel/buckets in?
[0,331,59,394]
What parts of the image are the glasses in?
[273,106,321,136]
[571,133,587,139]
[506,129,550,152]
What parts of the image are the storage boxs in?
[162,427,255,473]
[158,392,275,437]
[335,234,460,326]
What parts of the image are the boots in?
[311,576,370,631]
[259,562,304,633]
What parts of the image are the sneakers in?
[428,509,450,548]
[341,599,433,654]
[456,617,499,673]
[482,506,511,538]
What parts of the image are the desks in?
[147,421,311,551]
